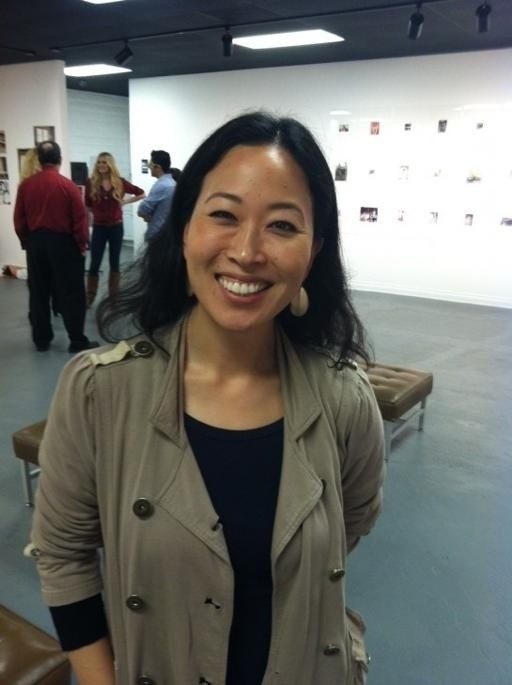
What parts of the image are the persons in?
[12,141,182,353]
[24,114,386,685]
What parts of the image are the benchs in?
[354,358,432,454]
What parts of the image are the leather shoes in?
[33,341,53,352]
[67,341,97,354]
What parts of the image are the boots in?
[109,272,122,305]
[86,274,99,308]
